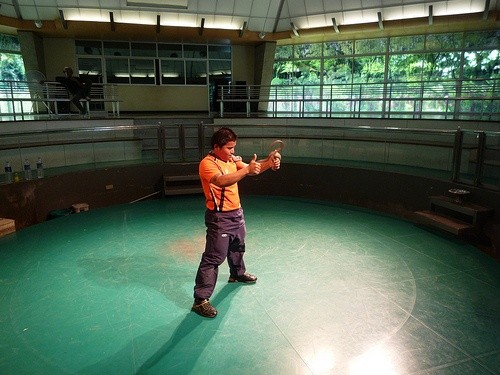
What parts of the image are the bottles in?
[36,157,44,178]
[24,159,33,180]
[4,160,12,181]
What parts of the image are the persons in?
[55,66,92,115]
[192,126,281,318]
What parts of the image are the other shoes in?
[228,271,257,284]
[193,300,217,318]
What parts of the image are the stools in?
[72,203,89,213]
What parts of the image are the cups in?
[14,172,20,181]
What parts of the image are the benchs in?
[0,80,123,119]
[218,78,500,130]
